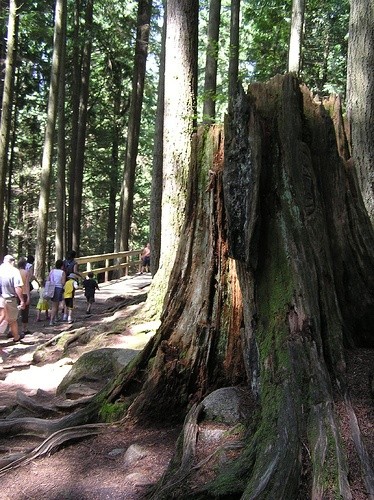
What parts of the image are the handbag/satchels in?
[42,269,54,299]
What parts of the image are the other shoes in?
[45,316,50,320]
[86,310,90,314]
[62,317,73,324]
[36,317,40,322]
[49,317,60,326]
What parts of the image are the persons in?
[0,254,25,342]
[141,243,150,274]
[48,259,66,326]
[37,280,50,322]
[0,283,7,363]
[62,273,79,324]
[7,256,32,338]
[19,255,41,318]
[81,272,100,314]
[62,250,86,314]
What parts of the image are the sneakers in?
[6,332,12,338]
[21,329,31,335]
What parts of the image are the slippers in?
[13,334,25,342]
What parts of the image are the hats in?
[67,273,79,280]
